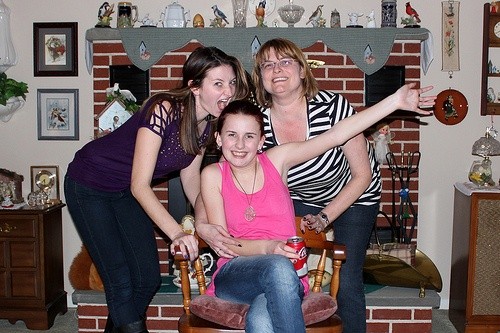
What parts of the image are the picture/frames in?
[33,22,79,76]
[37,88,79,141]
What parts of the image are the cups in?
[28,189,48,208]
[331,8,341,28]
[230,0,250,29]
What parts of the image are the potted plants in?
[0,72,29,122]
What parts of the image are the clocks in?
[31,165,60,204]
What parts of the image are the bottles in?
[380,0,399,29]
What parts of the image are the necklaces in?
[229,163,257,221]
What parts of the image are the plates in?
[432,89,468,125]
[173,277,212,289]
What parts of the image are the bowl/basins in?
[278,1,305,29]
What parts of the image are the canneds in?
[286,236,308,278]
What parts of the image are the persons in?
[199,81,438,333]
[195,39,381,332]
[63,47,249,333]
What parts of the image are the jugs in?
[117,1,139,29]
[159,1,190,28]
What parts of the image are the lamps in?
[471,127,500,187]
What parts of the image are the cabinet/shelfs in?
[480,2,500,116]
[0,201,67,330]
[448,183,500,333]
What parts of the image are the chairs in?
[174,217,347,333]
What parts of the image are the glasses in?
[259,59,297,70]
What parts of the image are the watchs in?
[318,211,330,226]
